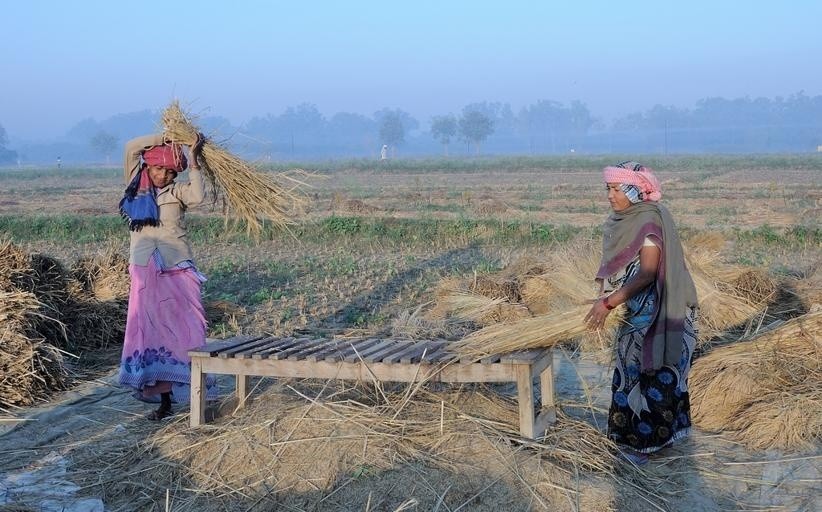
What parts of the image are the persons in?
[118,132,217,423]
[581,161,698,466]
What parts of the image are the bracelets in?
[188,164,200,169]
[603,296,615,310]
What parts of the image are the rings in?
[200,138,204,141]
[596,319,600,324]
